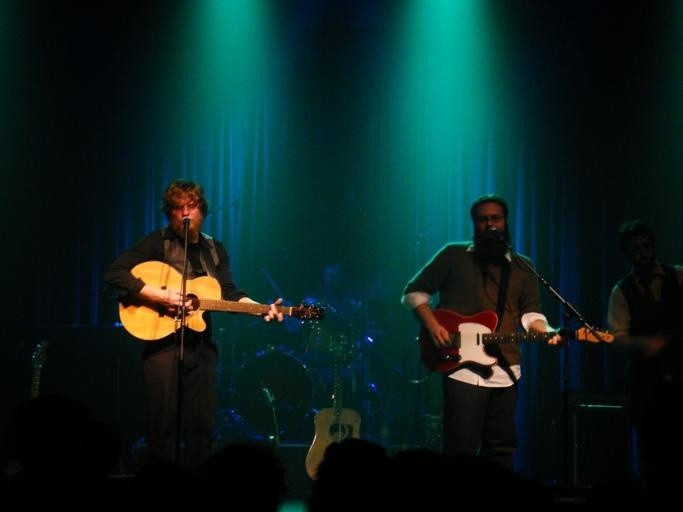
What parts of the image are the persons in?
[2,407,289,510]
[294,264,369,410]
[401,196,563,456]
[103,180,284,448]
[307,437,646,510]
[607,218,682,480]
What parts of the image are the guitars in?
[306,336,361,480]
[119,260,324,342]
[31,342,50,399]
[262,387,282,448]
[419,310,614,373]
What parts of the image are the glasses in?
[474,215,505,221]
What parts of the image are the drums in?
[235,347,327,434]
[302,298,352,362]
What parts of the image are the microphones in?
[183,217,190,232]
[488,227,512,249]
